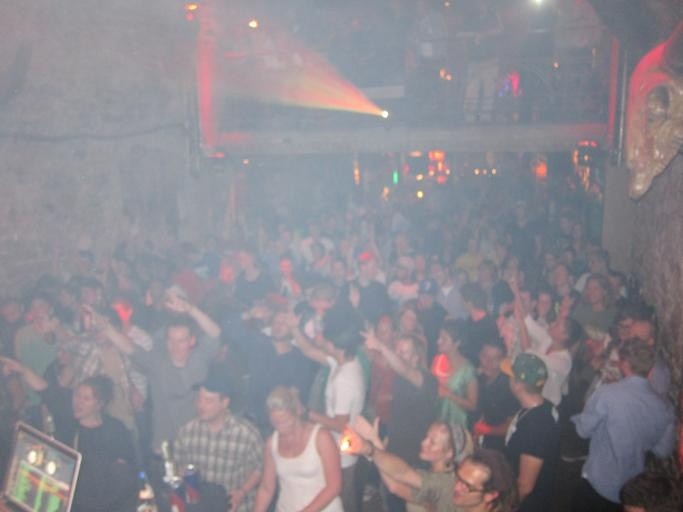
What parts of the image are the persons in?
[1,172,683,510]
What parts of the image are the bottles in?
[159,441,177,490]
[136,470,160,512]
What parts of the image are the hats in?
[500,352,547,386]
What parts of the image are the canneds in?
[169,476,184,512]
[184,463,201,504]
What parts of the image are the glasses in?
[455,471,493,490]
